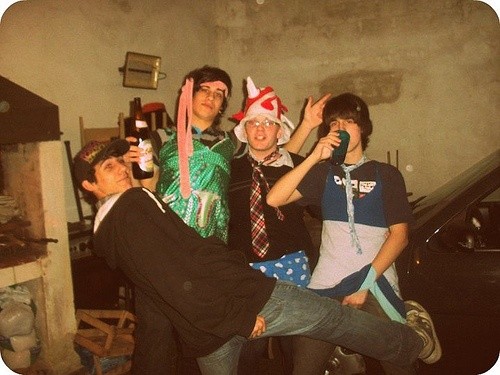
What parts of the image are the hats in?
[73,138,130,194]
[232,75,295,145]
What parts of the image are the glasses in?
[246,119,277,128]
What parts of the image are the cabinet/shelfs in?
[0,75,84,375]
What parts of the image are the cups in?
[330,130,350,165]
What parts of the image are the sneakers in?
[402,300,443,365]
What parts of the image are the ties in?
[247,148,285,258]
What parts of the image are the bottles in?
[130,97,154,179]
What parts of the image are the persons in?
[121,65,332,375]
[264,93,410,375]
[230,76,322,375]
[70,137,442,375]
